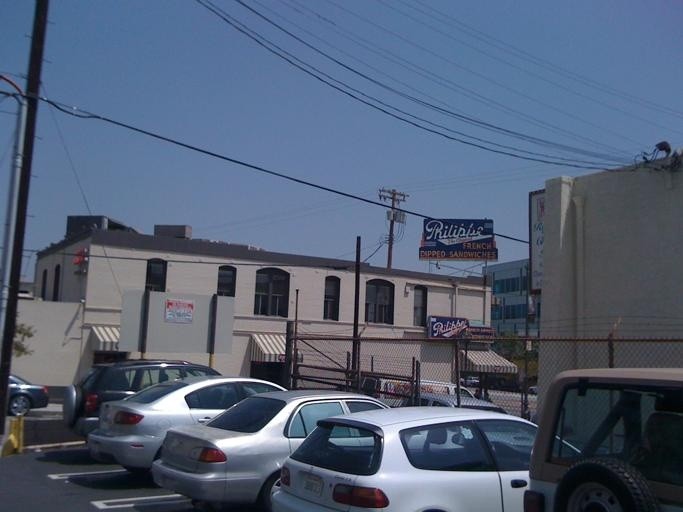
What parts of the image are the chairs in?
[644,413,683,483]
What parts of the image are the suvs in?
[60,357,229,440]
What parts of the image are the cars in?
[6,374,50,418]
